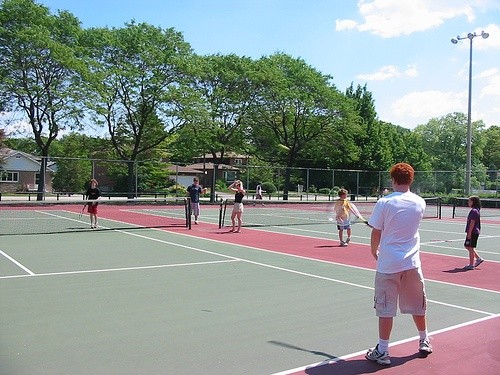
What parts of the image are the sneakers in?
[419,339,433,352]
[346,237,350,243]
[465,264,475,269]
[340,241,348,246]
[475,257,484,267]
[365,344,391,365]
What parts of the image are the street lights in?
[450,30,489,195]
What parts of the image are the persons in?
[253,183,264,206]
[464,196,484,270]
[86,179,101,228]
[227,180,245,233]
[335,189,362,247]
[185,178,207,225]
[366,163,432,366]
